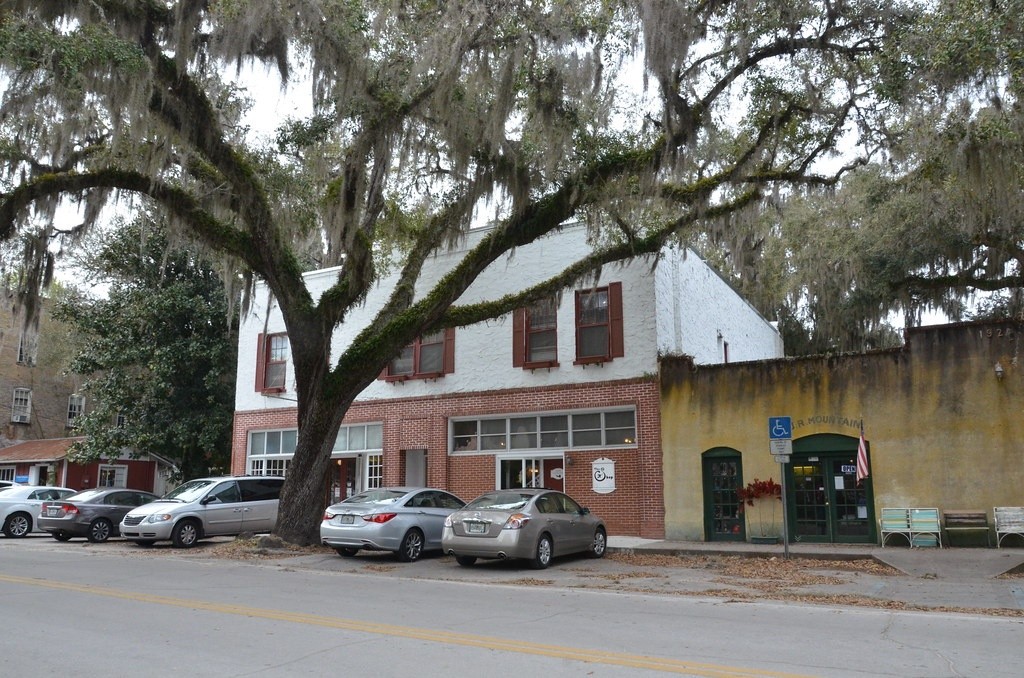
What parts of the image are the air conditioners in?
[15,415,28,422]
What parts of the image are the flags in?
[856,432,869,486]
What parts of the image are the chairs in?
[420,499,432,506]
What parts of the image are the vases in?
[750,536,779,545]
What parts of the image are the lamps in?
[565,455,574,466]
[995,366,1005,382]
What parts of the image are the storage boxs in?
[912,534,936,540]
[912,540,937,546]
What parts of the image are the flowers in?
[736,478,782,536]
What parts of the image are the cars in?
[319,487,471,562]
[0,484,80,538]
[0,481,22,496]
[441,488,608,569]
[36,487,163,543]
[119,473,291,548]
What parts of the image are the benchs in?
[879,507,942,549]
[993,506,1024,548]
[943,510,992,548]
